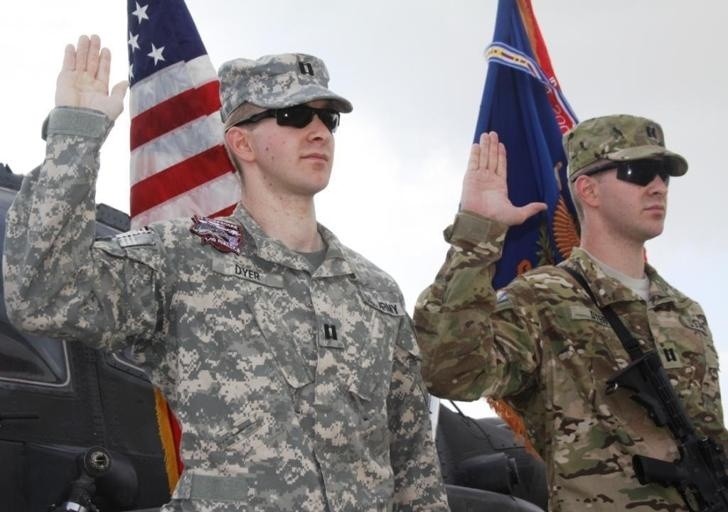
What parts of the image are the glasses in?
[225,102,342,135]
[571,156,671,187]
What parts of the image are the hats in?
[562,114,688,177]
[217,51,353,123]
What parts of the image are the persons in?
[413,107,727,512]
[3,30,457,512]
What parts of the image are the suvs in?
[0,161,554,512]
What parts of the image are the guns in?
[607,352,728,510]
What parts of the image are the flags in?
[117,0,245,507]
[464,0,586,465]
